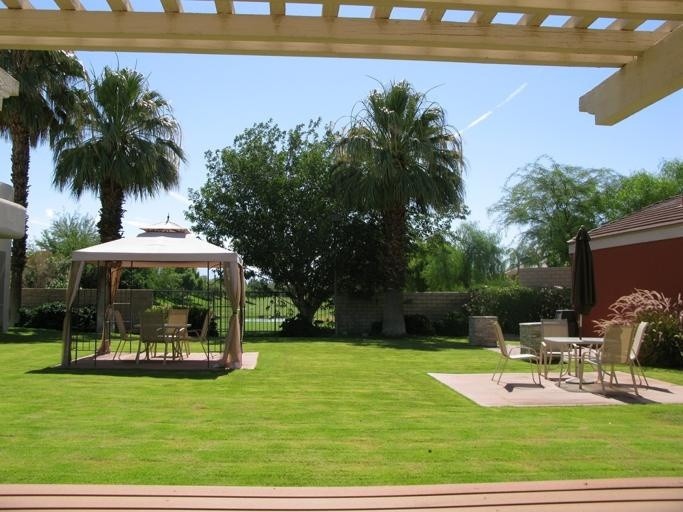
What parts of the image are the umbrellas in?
[571,224,596,363]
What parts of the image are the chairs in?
[112,308,214,368]
[490,318,649,398]
